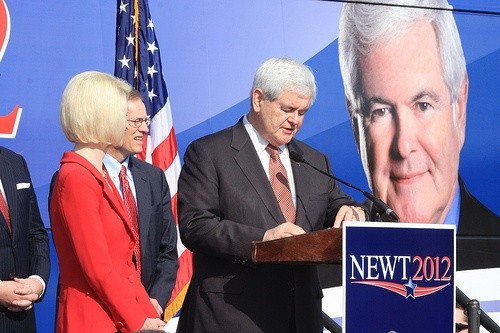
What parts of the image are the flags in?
[112,0,195,323]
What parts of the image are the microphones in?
[289,151,400,221]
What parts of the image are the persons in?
[173,57,374,333]
[337,0,500,270]
[0,147,50,333]
[49,71,167,333]
[50,87,180,333]
[456,303,468,333]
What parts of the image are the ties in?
[266,143,296,225]
[0,190,11,233]
[119,166,141,283]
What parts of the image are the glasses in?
[126,115,152,127]
